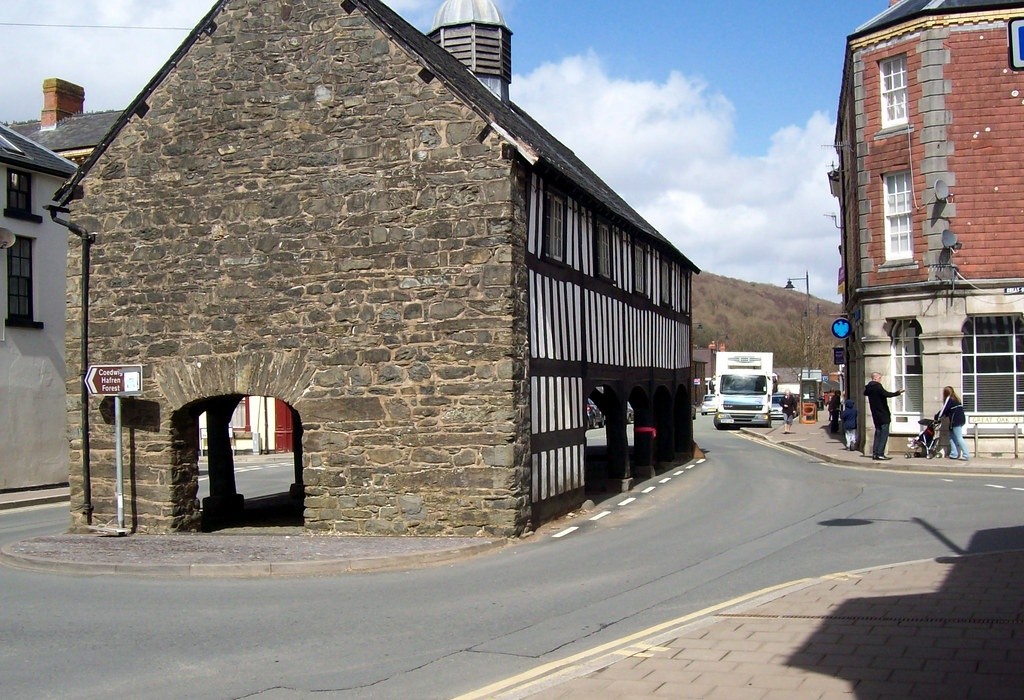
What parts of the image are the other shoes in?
[948,455,958,459]
[873,455,876,460]
[876,456,892,460]
[907,444,914,449]
[959,457,970,461]
[908,437,913,443]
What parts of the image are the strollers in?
[903,419,943,460]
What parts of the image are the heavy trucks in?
[714,351,779,430]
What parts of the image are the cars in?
[769,393,786,420]
[586,398,605,431]
[626,402,634,425]
[701,395,716,416]
[691,399,697,419]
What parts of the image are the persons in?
[828,390,841,434]
[840,400,857,451]
[906,425,927,449]
[779,389,797,434]
[863,372,905,460]
[934,386,969,460]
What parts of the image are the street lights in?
[784,271,811,371]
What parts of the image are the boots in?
[784,423,788,434]
[787,425,791,434]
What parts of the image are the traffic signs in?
[85,362,145,397]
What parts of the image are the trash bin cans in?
[817,398,823,411]
